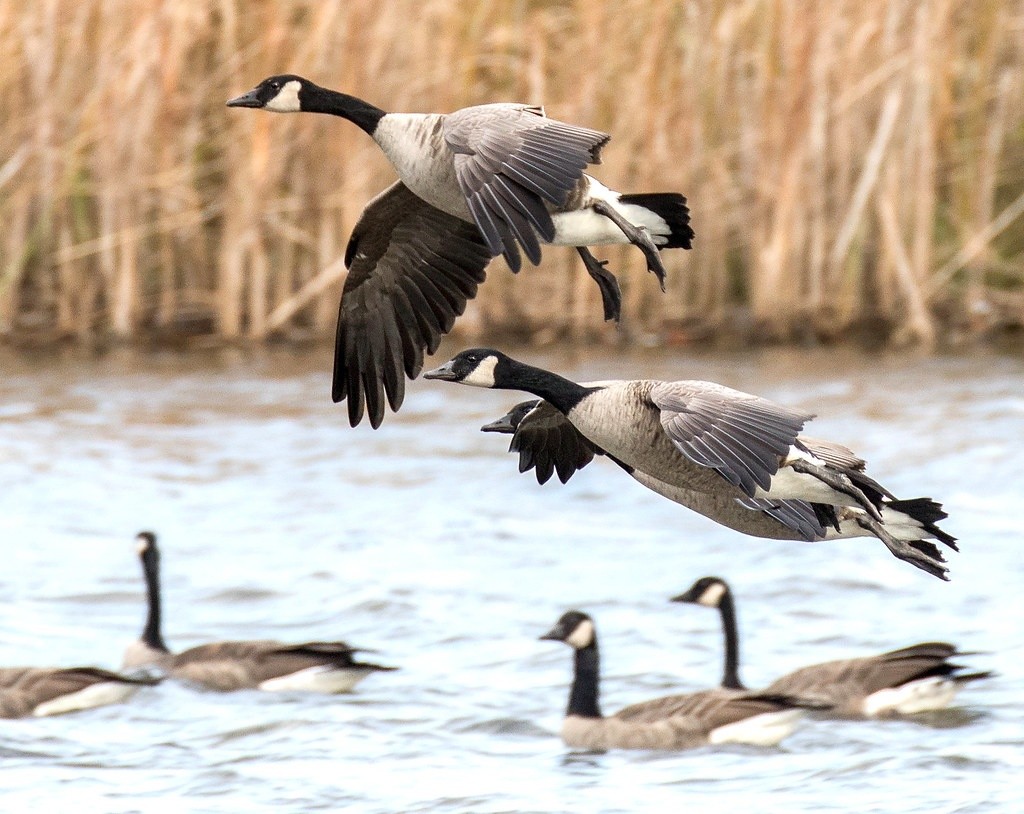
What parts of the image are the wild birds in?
[0,532,402,719]
[538,577,1008,748]
[225,73,962,583]
